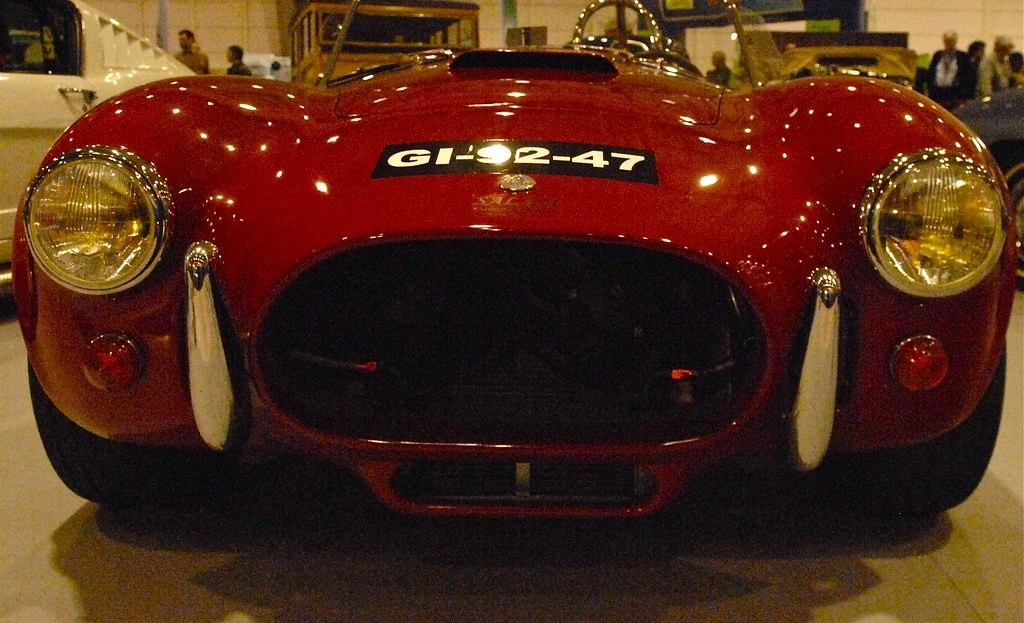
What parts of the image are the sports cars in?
[14,2,1010,518]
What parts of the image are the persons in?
[664,31,1024,110]
[174,29,289,82]
